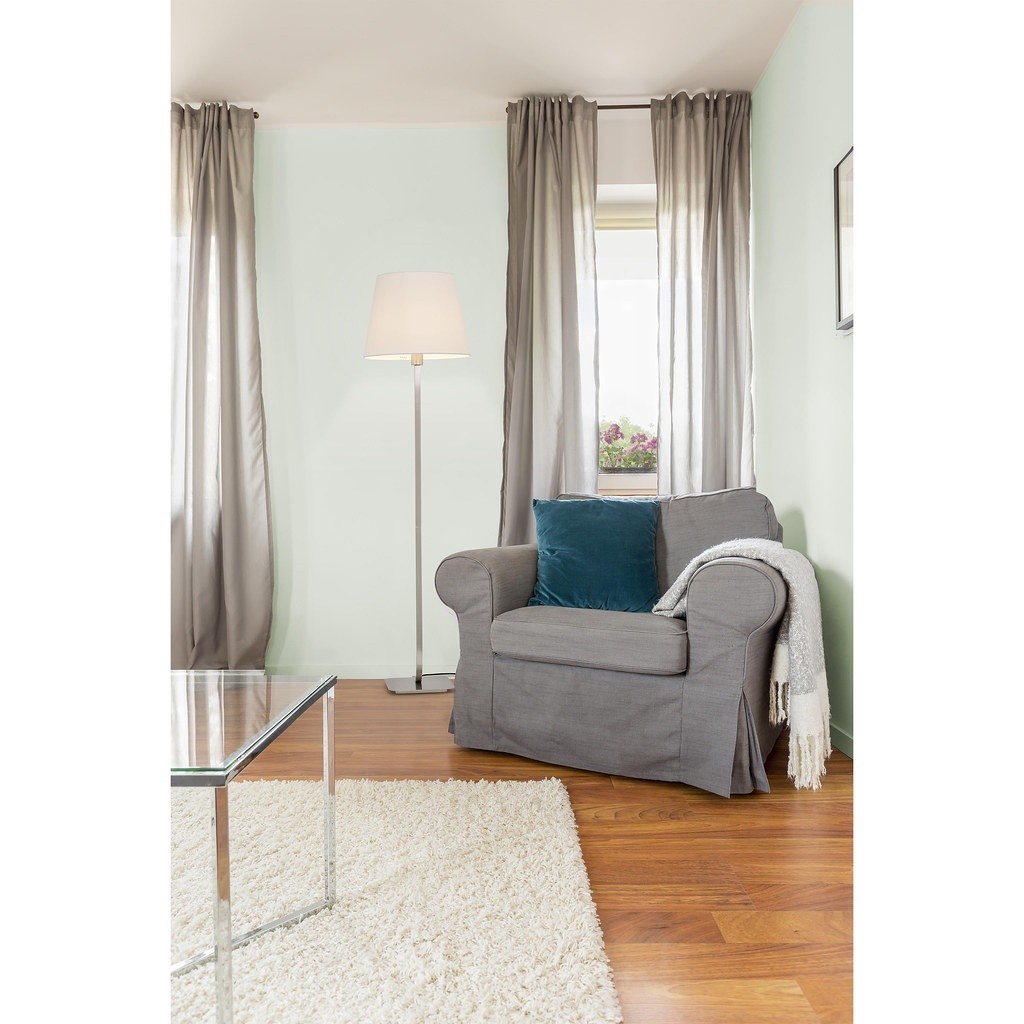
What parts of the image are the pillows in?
[525,497,664,612]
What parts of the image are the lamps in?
[364,269,474,695]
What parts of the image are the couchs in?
[434,486,837,799]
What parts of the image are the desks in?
[169,670,339,1024]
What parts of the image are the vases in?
[600,465,657,473]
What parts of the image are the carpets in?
[169,776,622,1024]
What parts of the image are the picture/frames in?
[834,146,853,331]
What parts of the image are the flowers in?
[597,423,659,467]
